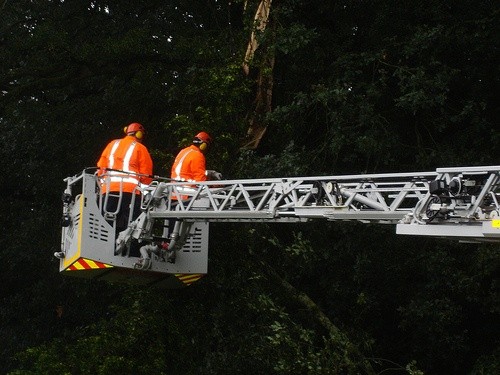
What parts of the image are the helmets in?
[124,123,145,135]
[195,132,210,146]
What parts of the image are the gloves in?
[209,170,222,180]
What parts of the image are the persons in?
[97,123,152,257]
[171,132,222,201]
